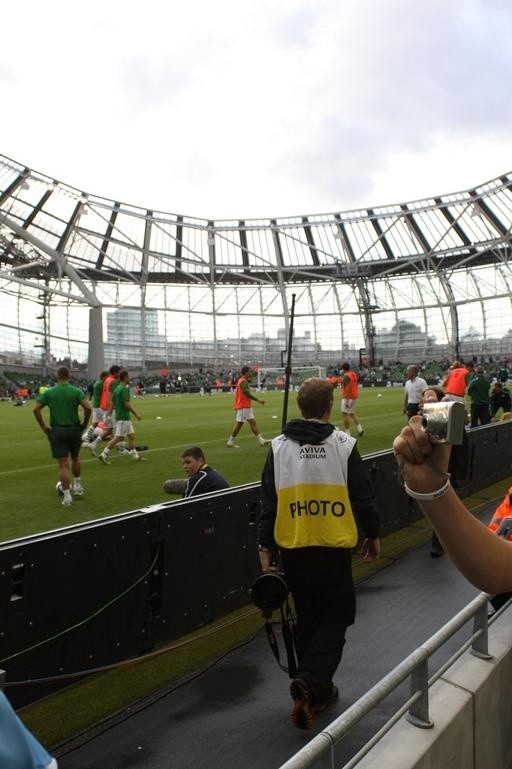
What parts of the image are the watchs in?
[255,544,270,552]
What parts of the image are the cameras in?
[249,553,290,612]
[421,402,467,445]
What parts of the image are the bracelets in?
[403,477,452,503]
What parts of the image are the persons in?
[442,362,473,432]
[81,371,109,447]
[341,362,366,437]
[467,366,491,427]
[418,388,474,557]
[181,446,230,498]
[34,368,92,507]
[87,365,131,456]
[99,370,148,464]
[391,415,512,596]
[402,365,428,421]
[82,414,111,440]
[228,366,270,449]
[257,377,382,730]
[490,382,512,417]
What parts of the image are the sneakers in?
[88,444,97,457]
[119,449,132,456]
[74,488,83,496]
[358,430,364,437]
[98,454,111,464]
[290,679,314,731]
[261,440,271,446]
[313,687,334,712]
[227,441,239,449]
[64,499,72,507]
